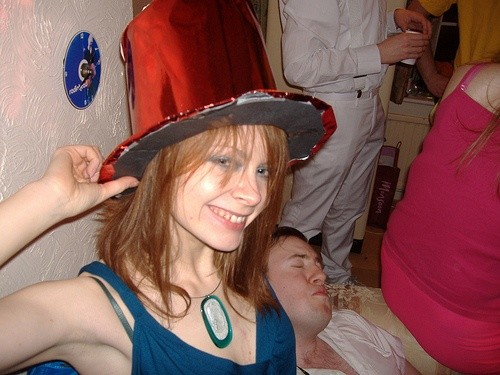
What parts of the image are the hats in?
[98,0,337,202]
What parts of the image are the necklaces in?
[189,278,233,348]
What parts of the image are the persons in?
[279,0,433,286]
[0,0,337,375]
[381,47,500,375]
[266,226,422,375]
[408,0,500,97]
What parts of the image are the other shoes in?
[345,277,366,288]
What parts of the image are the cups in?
[400,28,423,66]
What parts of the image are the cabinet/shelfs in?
[386,98,436,194]
